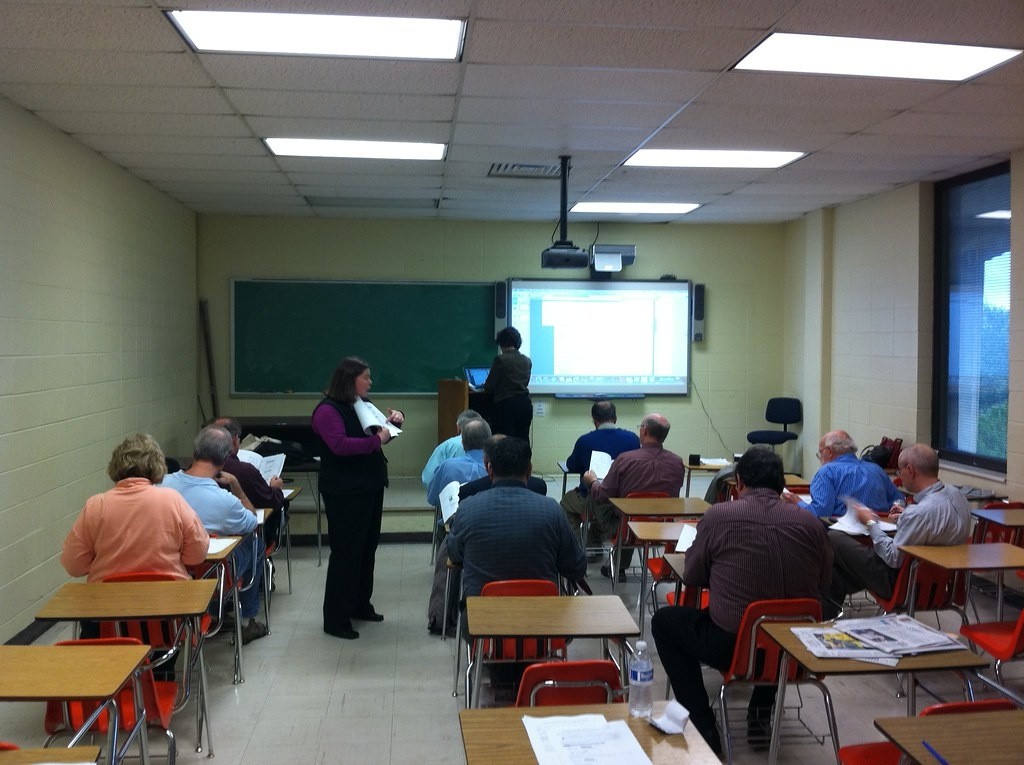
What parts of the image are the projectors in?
[542,248,588,268]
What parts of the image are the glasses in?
[893,463,908,476]
[816,446,829,458]
[637,425,647,430]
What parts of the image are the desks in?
[723,474,810,501]
[250,508,273,635]
[661,553,709,702]
[761,613,993,765]
[202,536,245,686]
[0,745,101,765]
[627,521,698,641]
[281,486,302,594]
[874,711,1024,765]
[465,595,641,709]
[458,701,724,765]
[35,579,220,758]
[969,508,1024,622]
[558,461,583,499]
[820,516,898,616]
[606,497,712,595]
[0,644,152,765]
[896,543,1024,694]
[681,460,731,497]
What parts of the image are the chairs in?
[746,397,802,453]
[0,472,1024,765]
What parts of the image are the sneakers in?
[232,617,266,646]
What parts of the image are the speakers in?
[692,283,705,340]
[495,280,508,339]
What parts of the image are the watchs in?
[865,519,877,530]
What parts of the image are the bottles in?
[629,641,655,719]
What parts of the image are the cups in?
[688,453,701,465]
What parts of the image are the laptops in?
[463,365,492,392]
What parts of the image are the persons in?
[825,443,971,620]
[62,434,209,682]
[150,426,267,644]
[214,418,283,593]
[480,327,533,443]
[311,357,405,639]
[650,446,832,756]
[782,430,904,522]
[420,400,685,656]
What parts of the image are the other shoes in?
[747,719,772,751]
[324,629,359,640]
[351,611,384,621]
[701,725,722,758]
[601,565,627,583]
